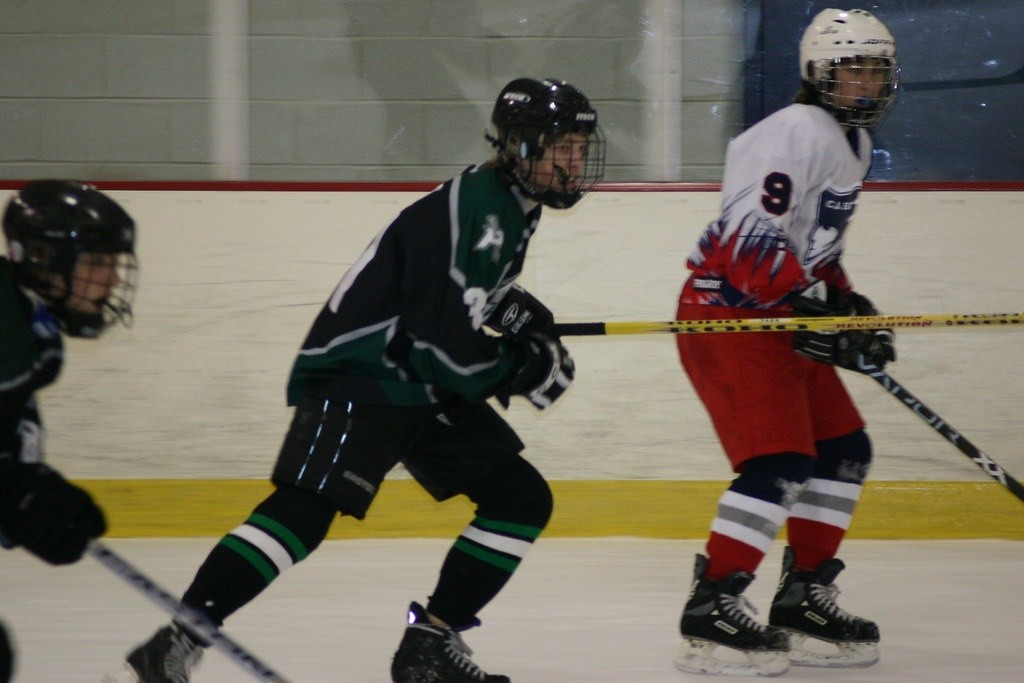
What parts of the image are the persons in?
[118,74,597,683]
[674,7,895,655]
[0,176,135,683]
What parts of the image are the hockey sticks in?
[88,539,290,683]
[532,311,1024,337]
[859,354,1024,501]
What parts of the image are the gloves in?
[854,295,895,367]
[481,281,555,334]
[519,331,575,409]
[792,305,863,364]
[3,458,107,565]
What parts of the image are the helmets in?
[2,178,138,339]
[491,76,605,208]
[800,6,901,129]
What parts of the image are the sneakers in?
[391,601,510,682]
[767,546,880,667]
[123,619,205,682]
[674,552,790,677]
